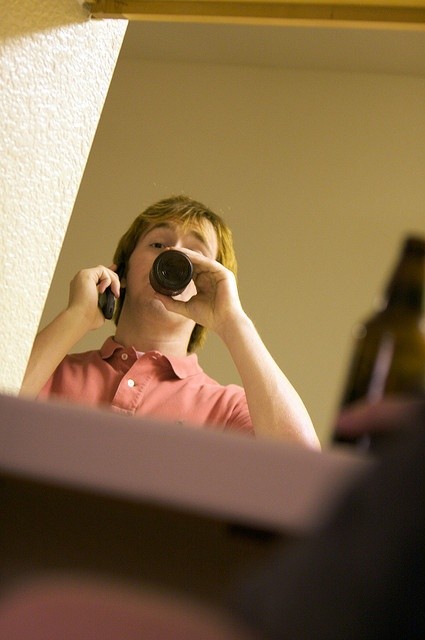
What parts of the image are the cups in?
[150,250,193,296]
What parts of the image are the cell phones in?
[97,283,117,320]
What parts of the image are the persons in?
[14,197,322,454]
